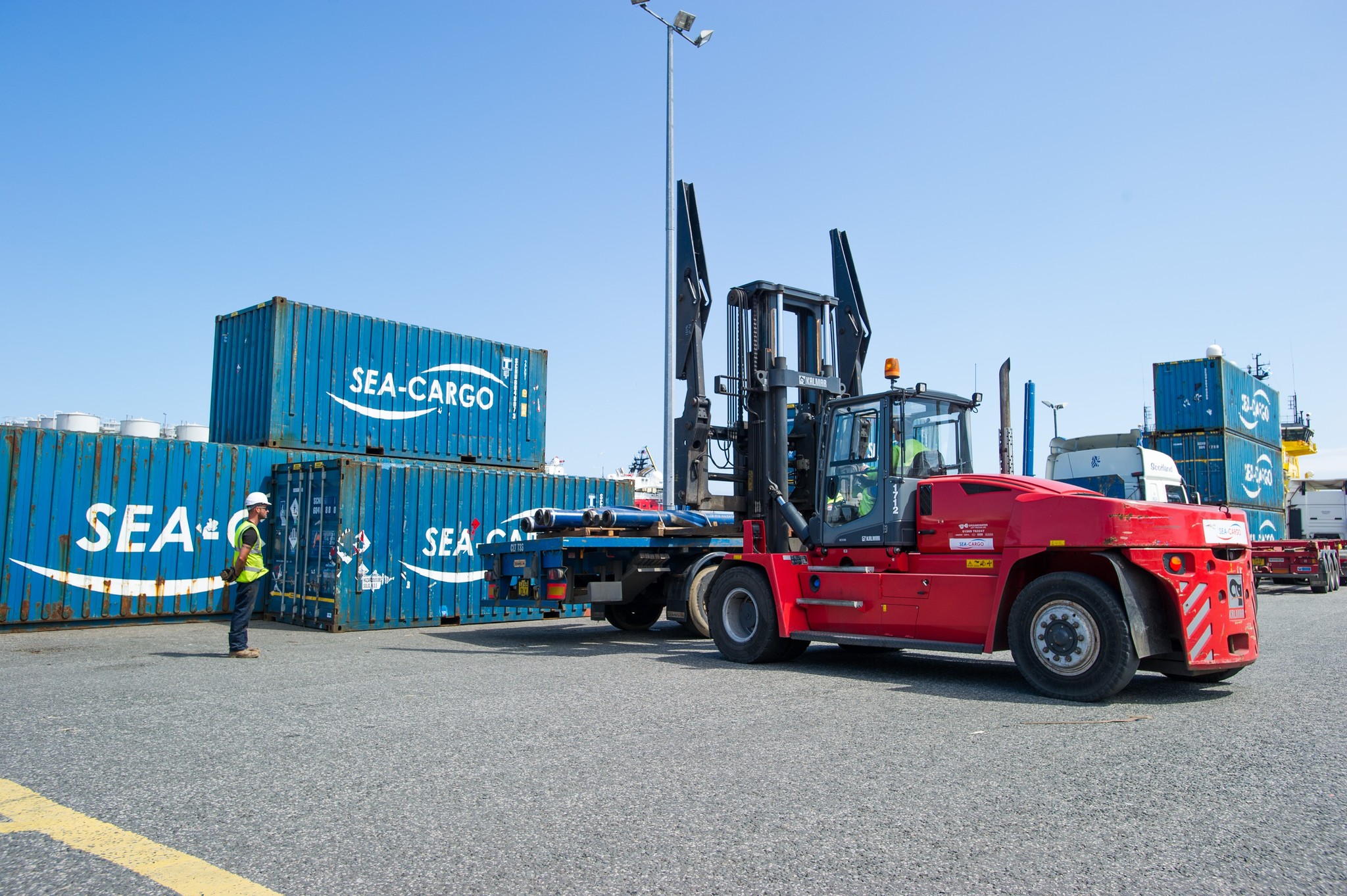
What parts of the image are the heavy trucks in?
[475,401,928,642]
[1246,478,1347,594]
[1052,446,1201,505]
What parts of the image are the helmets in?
[245,492,272,511]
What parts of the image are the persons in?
[221,492,271,658]
[857,419,931,519]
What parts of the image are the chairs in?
[905,450,947,478]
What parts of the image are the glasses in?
[257,506,269,511]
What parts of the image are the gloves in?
[220,566,238,583]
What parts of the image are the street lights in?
[1040,400,1064,437]
[631,0,714,510]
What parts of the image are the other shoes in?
[247,647,260,655]
[228,648,260,658]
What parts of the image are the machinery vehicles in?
[671,180,1259,705]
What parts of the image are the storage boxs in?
[209,296,548,469]
[1237,507,1288,540]
[265,458,635,632]
[1153,356,1281,451]
[0,425,546,631]
[787,403,939,492]
[1155,431,1286,512]
